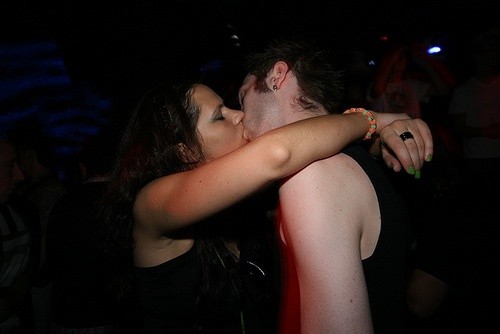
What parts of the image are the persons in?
[101,70,435,331]
[227,37,500,334]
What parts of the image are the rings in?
[398,131,415,144]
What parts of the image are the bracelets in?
[343,106,377,141]
